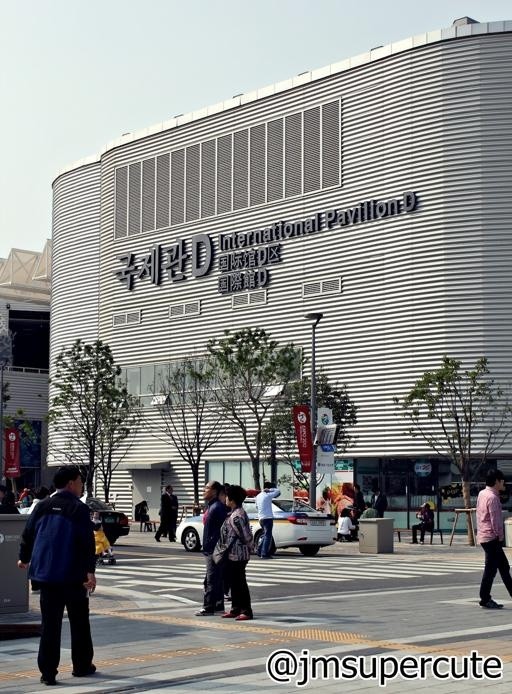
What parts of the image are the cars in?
[21,496,133,547]
[173,487,339,559]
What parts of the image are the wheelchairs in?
[94,547,117,566]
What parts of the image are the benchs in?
[390,526,445,544]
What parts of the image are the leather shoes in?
[221,611,240,617]
[234,613,253,620]
[480,600,505,610]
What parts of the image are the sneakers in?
[72,664,98,678]
[193,607,226,616]
[38,673,57,688]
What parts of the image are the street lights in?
[302,310,324,509]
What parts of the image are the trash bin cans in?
[0,514,32,614]
[504,517,512,548]
[357,518,394,554]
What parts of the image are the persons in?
[219,484,258,620]
[154,485,178,542]
[193,480,227,616]
[16,465,97,686]
[334,482,355,518]
[255,482,281,559]
[337,507,359,542]
[316,486,332,514]
[0,475,114,594]
[363,478,371,491]
[352,484,365,510]
[359,502,380,519]
[219,484,227,503]
[134,499,153,531]
[370,486,388,518]
[409,502,434,544]
[475,469,511,609]
